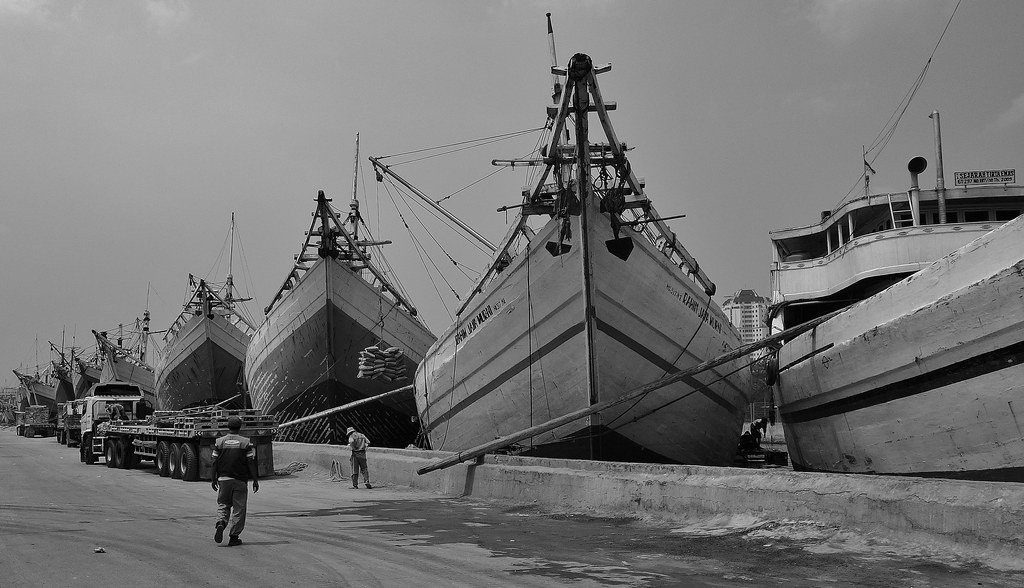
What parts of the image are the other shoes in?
[349,486,358,489]
[367,485,371,488]
[228,539,241,546]
[214,525,224,543]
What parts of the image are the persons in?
[344,426,371,489]
[211,417,259,546]
[105,403,129,425]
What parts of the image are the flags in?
[864,160,875,176]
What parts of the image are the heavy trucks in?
[16,405,55,439]
[79,385,274,483]
[56,398,85,448]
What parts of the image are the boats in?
[764,109,1024,487]
[242,134,439,446]
[9,279,161,415]
[153,213,257,417]
[410,14,758,467]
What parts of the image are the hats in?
[346,427,355,435]
